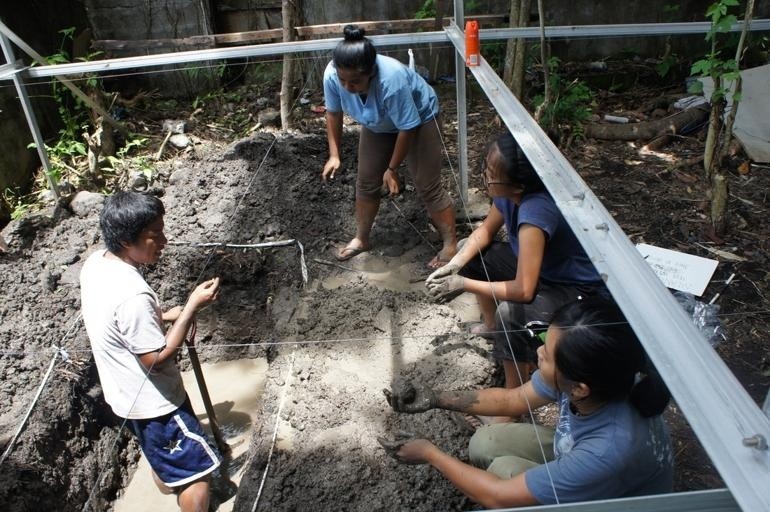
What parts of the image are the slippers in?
[335,242,372,261]
[426,252,450,270]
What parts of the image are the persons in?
[423,126,616,436]
[78,188,226,512]
[376,296,678,512]
[321,21,460,271]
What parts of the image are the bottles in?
[464,21,481,67]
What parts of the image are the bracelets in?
[388,165,395,171]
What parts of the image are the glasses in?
[481,159,514,188]
